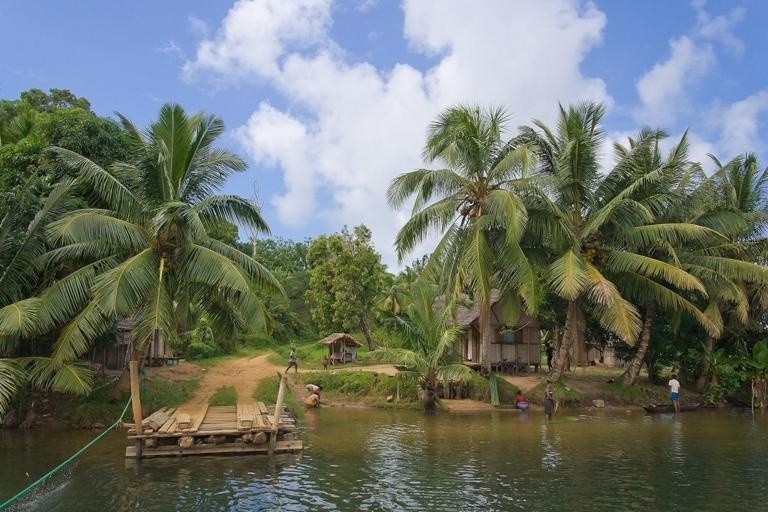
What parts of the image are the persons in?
[372,372,378,389]
[427,383,435,408]
[323,357,328,370]
[285,346,298,373]
[669,374,680,418]
[544,381,554,420]
[565,351,572,371]
[514,391,529,405]
[545,344,554,373]
[305,384,321,408]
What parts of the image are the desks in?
[145,356,184,367]
